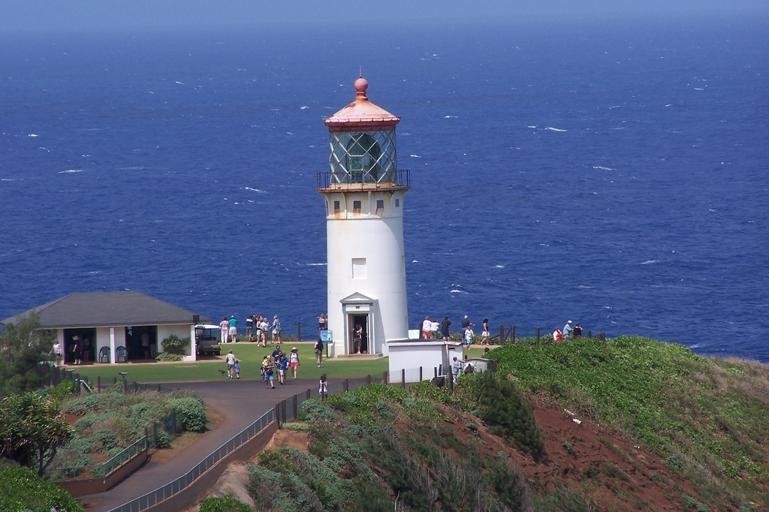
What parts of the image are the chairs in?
[99,346,110,363]
[116,346,128,362]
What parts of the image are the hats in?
[291,346,298,351]
[230,314,278,322]
[72,334,79,341]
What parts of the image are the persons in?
[573,324,583,336]
[220,316,229,343]
[421,314,491,350]
[315,338,323,366]
[228,314,237,343]
[260,344,301,389]
[73,335,81,365]
[319,312,325,330]
[225,349,235,377]
[49,339,63,364]
[563,319,573,336]
[552,328,561,343]
[234,360,241,378]
[317,374,329,396]
[245,313,282,348]
